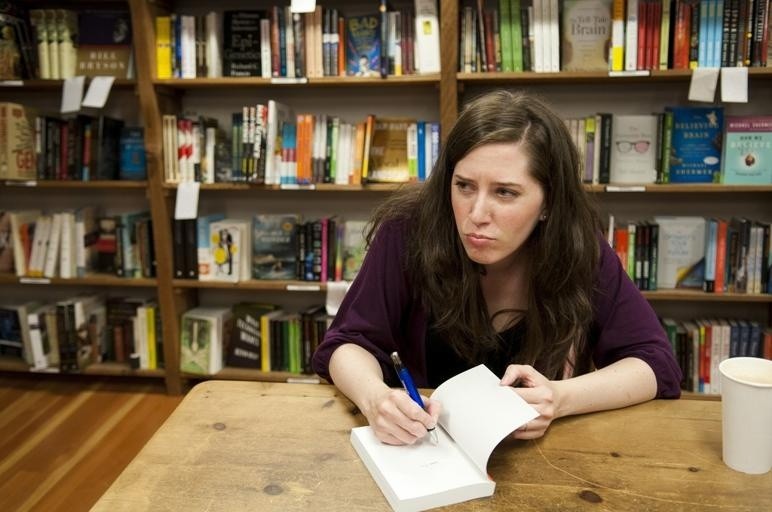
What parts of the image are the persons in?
[311,87,683,448]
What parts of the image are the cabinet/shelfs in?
[0,2,771,397]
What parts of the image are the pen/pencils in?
[391,352,439,445]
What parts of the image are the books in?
[460,0,772,72]
[658,315,772,393]
[563,106,772,185]
[163,100,442,185]
[0,102,149,180]
[3,4,137,80]
[346,362,542,511]
[0,291,165,374]
[603,212,772,294]
[155,0,442,80]
[180,302,336,374]
[0,204,157,278]
[170,212,376,282]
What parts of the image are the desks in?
[89,380,770,511]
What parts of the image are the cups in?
[717,356,772,475]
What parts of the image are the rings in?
[525,422,530,432]
